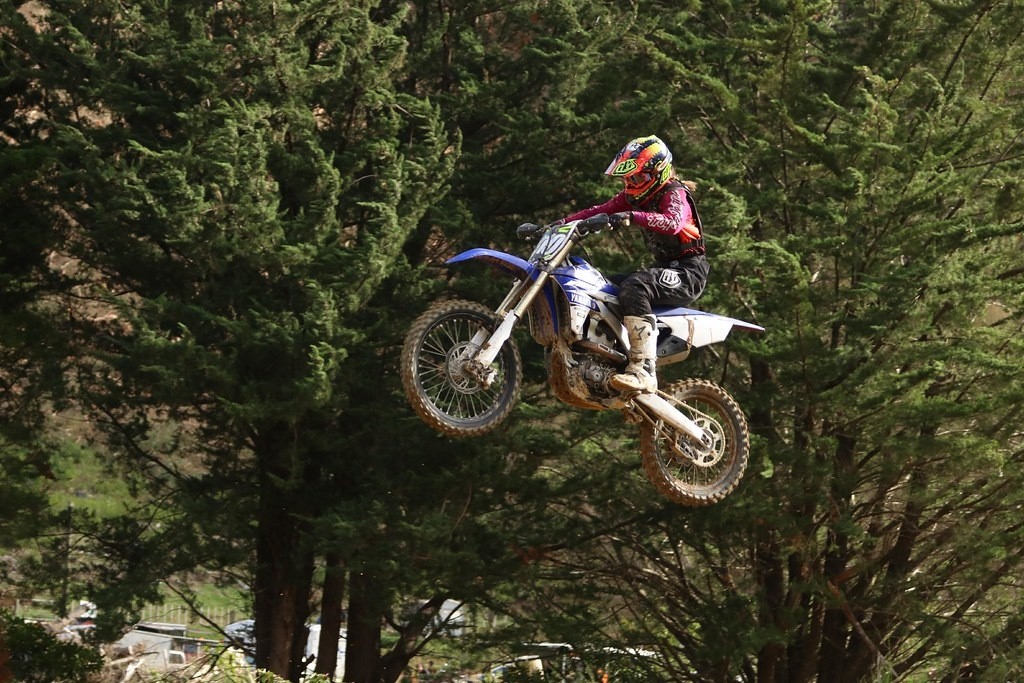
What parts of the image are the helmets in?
[605,136,674,206]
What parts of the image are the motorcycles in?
[401,213,767,506]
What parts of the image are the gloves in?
[517,223,541,240]
[609,211,634,232]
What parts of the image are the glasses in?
[625,173,651,188]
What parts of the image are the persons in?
[535,135,708,394]
[418,660,437,683]
[482,662,492,683]
[544,659,553,680]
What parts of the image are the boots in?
[611,313,658,394]
